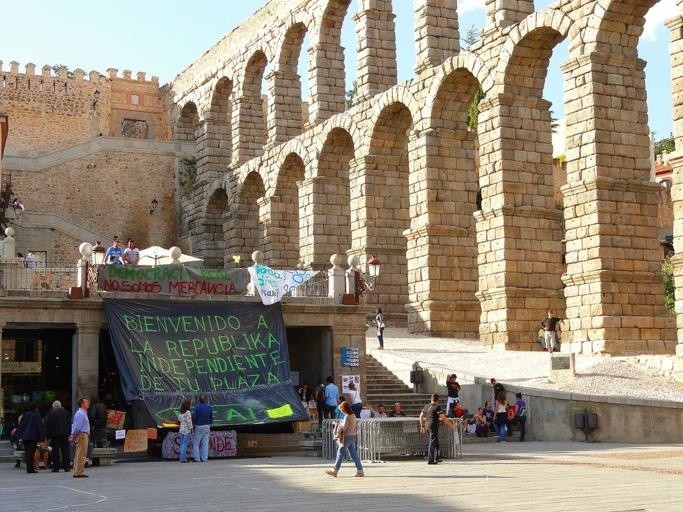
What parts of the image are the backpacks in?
[518,400,527,417]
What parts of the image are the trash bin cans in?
[410,369,423,384]
[575,413,598,429]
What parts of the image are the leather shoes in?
[27,458,92,478]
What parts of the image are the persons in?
[326,401,364,479]
[541,311,563,352]
[296,374,528,443]
[92,238,141,266]
[9,396,119,479]
[192,394,213,463]
[374,307,386,350]
[16,251,25,268]
[178,399,194,463]
[538,322,547,349]
[26,252,36,268]
[420,394,458,465]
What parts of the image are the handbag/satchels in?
[467,423,477,434]
[377,329,382,337]
[377,320,385,329]
[506,406,517,422]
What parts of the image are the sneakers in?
[353,471,364,477]
[325,469,338,478]
[428,459,443,465]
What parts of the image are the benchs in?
[11,447,118,468]
